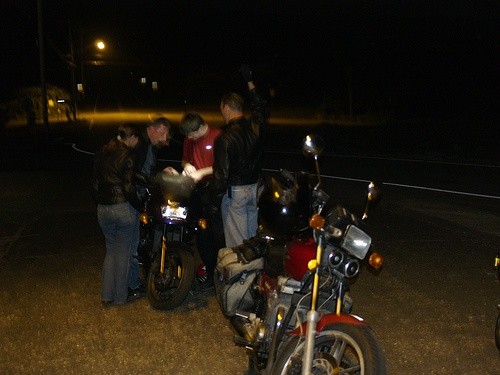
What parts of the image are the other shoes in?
[195,281,214,291]
[127,295,137,303]
[102,301,113,305]
[137,279,146,289]
[128,289,146,297]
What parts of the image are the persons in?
[93,124,162,304]
[131,117,183,299]
[213,70,268,249]
[181,112,223,287]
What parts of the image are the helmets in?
[256,172,314,237]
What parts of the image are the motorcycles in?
[137,172,206,311]
[193,133,385,375]
